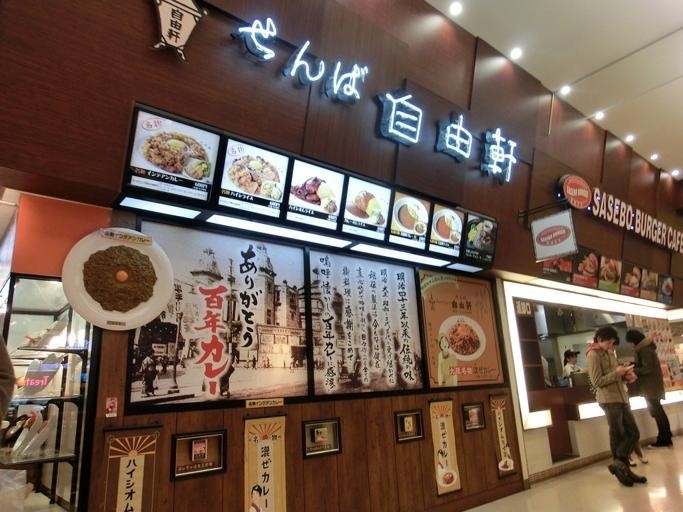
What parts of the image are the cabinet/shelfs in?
[0,271,90,512]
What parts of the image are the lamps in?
[534,304,549,342]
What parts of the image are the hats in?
[564,349,580,358]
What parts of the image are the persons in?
[610,336,649,465]
[624,328,674,449]
[437,448,449,476]
[435,333,459,388]
[136,350,159,397]
[247,484,265,512]
[562,349,584,379]
[583,326,647,486]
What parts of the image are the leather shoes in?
[608,463,634,487]
[627,468,646,484]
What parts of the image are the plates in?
[438,316,486,362]
[10,413,59,457]
[438,470,457,487]
[135,129,496,254]
[61,228,174,330]
[499,459,514,472]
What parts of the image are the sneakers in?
[628,456,636,466]
[635,445,648,464]
[647,442,674,450]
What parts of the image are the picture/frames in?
[169,429,227,482]
[393,408,425,444]
[302,417,342,460]
[461,402,486,433]
